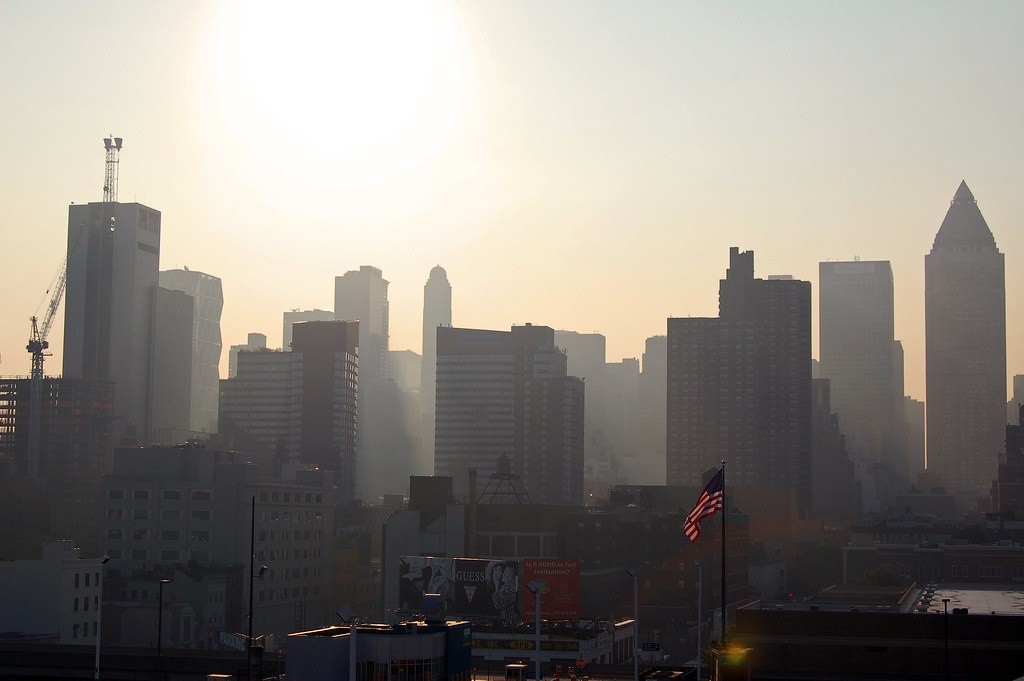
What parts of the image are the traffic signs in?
[576,658,586,668]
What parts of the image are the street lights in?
[336,606,356,681]
[691,559,702,681]
[157,579,171,655]
[526,581,541,681]
[624,568,638,681]
[95,555,110,678]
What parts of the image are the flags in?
[681,465,723,544]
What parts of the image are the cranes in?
[25,220,88,477]
